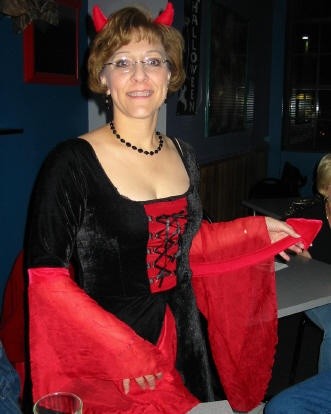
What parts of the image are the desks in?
[242,196,311,220]
[275,255,331,319]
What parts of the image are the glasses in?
[104,56,166,73]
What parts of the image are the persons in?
[21,3,322,414]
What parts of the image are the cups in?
[33,392,83,414]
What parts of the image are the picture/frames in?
[22,0,82,86]
[204,0,250,138]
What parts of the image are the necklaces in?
[109,119,164,155]
